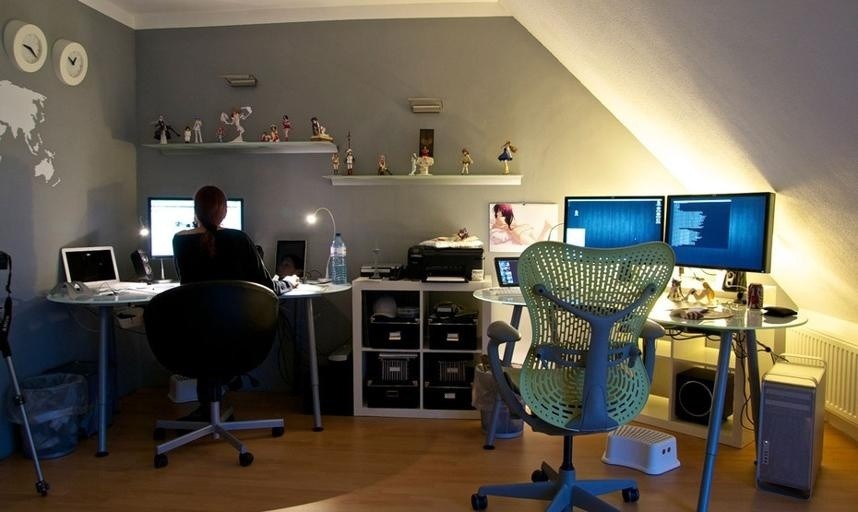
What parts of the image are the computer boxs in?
[755,353,827,502]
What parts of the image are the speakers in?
[675,367,734,427]
[130,249,153,285]
[274,239,307,280]
[255,245,264,260]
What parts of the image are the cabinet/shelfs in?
[633,330,786,449]
[350,274,493,422]
[142,137,525,188]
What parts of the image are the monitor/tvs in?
[147,196,246,260]
[662,192,775,299]
[562,196,666,282]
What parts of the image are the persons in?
[490,204,553,245]
[174,185,303,423]
[330,140,519,176]
[152,105,335,144]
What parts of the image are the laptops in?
[61,246,147,294]
[494,257,536,295]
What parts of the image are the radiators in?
[785,325,858,426]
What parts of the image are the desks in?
[42,277,352,457]
[471,286,811,510]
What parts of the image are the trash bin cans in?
[10,373,85,459]
[475,363,526,439]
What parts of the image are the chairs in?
[472,239,677,511]
[143,278,288,467]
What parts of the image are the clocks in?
[52,36,89,89]
[3,17,49,77]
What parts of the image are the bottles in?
[331,231,347,284]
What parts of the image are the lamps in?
[307,205,336,283]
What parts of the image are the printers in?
[407,245,484,283]
